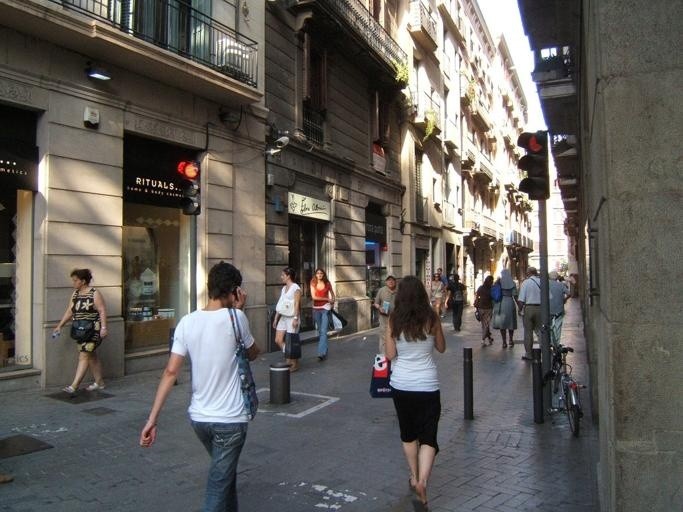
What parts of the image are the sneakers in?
[509,342,514,347]
[503,344,507,348]
[481,340,486,345]
[287,353,328,373]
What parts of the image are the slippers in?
[61,385,80,393]
[408,476,416,492]
[410,494,431,512]
[86,383,105,390]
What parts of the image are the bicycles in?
[538,311,588,437]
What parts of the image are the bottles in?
[52,330,61,339]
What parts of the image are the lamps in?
[85,65,112,80]
[219,112,240,123]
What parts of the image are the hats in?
[384,274,397,280]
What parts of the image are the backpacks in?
[450,283,463,305]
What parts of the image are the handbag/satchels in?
[490,281,502,302]
[235,348,260,421]
[70,319,95,341]
[285,328,301,360]
[328,303,348,331]
[369,356,395,399]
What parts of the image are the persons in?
[272,267,302,373]
[53,269,108,393]
[138,262,260,511]
[383,274,447,511]
[310,268,335,361]
[430,267,578,360]
[374,273,399,358]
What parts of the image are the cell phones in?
[231,287,238,302]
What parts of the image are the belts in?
[525,304,540,306]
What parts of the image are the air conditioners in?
[215,38,248,73]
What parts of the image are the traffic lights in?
[517,130,549,200]
[177,158,201,216]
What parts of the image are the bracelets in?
[101,327,107,329]
[146,419,158,427]
[293,316,298,320]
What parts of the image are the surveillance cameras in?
[274,135,290,149]
